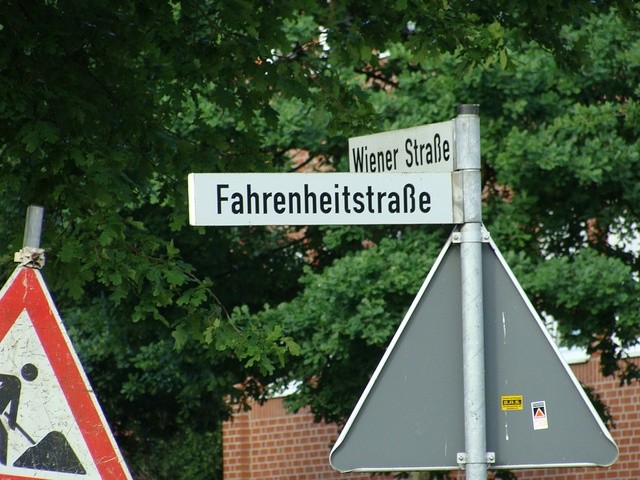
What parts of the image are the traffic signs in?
[348,121,453,172]
[188,174,452,226]
